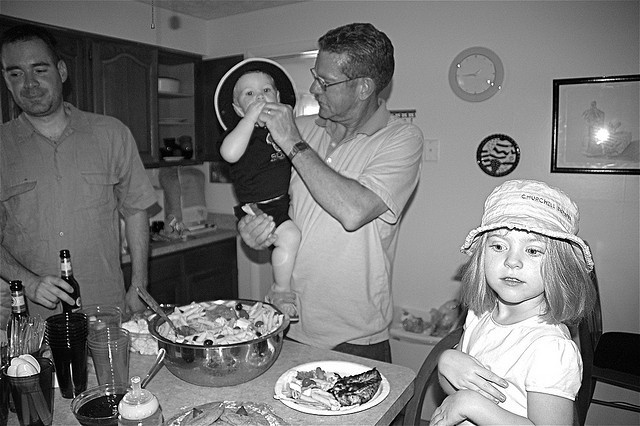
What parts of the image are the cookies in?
[179,401,271,426]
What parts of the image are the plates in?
[161,118,188,122]
[274,360,391,417]
[161,157,186,161]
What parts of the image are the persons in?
[428,179,598,426]
[258,22,425,364]
[0,21,160,348]
[214,57,300,322]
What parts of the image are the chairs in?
[569,239,639,426]
[390,319,464,426]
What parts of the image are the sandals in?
[265,281,299,322]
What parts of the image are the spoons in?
[10,358,30,425]
[16,363,50,425]
[136,286,199,342]
[6,365,16,377]
[23,354,52,421]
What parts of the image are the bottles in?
[7,280,30,346]
[59,249,82,312]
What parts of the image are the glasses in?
[310,67,367,91]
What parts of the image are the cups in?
[44,312,90,398]
[89,328,130,386]
[79,305,123,328]
[0,357,54,426]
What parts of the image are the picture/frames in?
[550,75,640,174]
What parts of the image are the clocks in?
[447,46,503,102]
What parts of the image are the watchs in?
[288,139,312,161]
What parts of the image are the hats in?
[214,56,298,131]
[461,178,594,273]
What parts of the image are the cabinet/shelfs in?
[83,31,245,169]
[2,13,83,124]
[121,236,238,308]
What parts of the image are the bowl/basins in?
[70,382,145,426]
[148,298,290,388]
